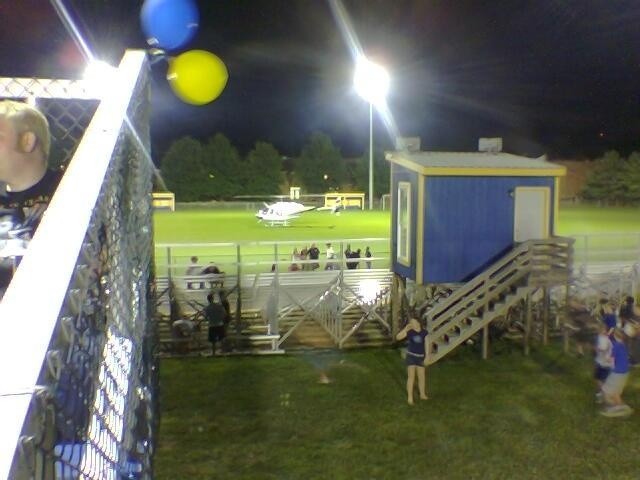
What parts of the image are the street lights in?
[361,67,381,210]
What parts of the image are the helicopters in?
[232,189,348,231]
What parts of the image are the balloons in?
[166,49,231,106]
[139,0,203,52]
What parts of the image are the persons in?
[202,293,230,359]
[216,290,232,326]
[0,99,65,270]
[394,315,434,407]
[570,294,640,419]
[183,256,203,289]
[202,262,221,287]
[287,241,373,272]
